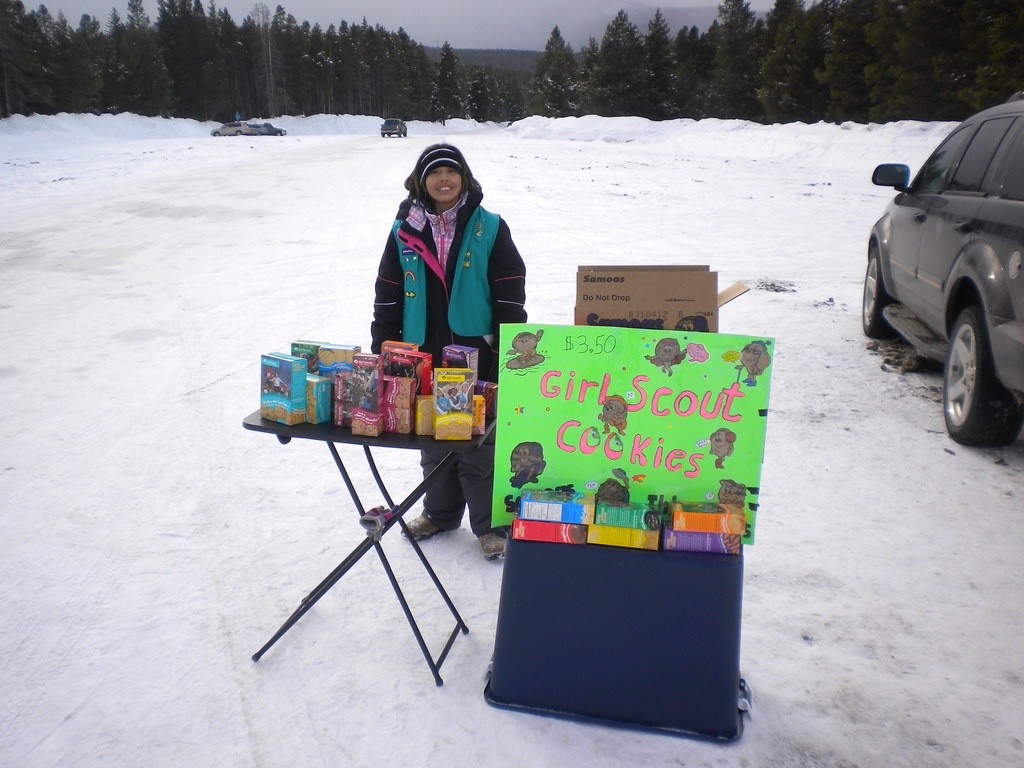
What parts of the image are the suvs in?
[861,92,1024,448]
[211,122,250,135]
[247,124,268,135]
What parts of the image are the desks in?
[241,409,498,686]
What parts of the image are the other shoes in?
[400,514,445,542]
[479,532,506,561]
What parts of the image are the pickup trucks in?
[264,122,287,137]
[380,118,407,137]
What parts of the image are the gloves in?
[360,505,401,541]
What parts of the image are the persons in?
[437,382,473,415]
[264,367,289,398]
[371,144,527,561]
[384,353,390,374]
[353,364,378,412]
[406,357,421,390]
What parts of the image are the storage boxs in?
[574,265,749,333]
[483,543,754,745]
[260,341,498,440]
[511,490,747,556]
